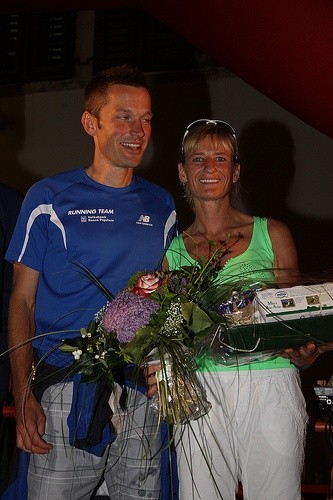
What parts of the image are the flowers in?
[1,220,332,500]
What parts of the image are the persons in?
[144,119,333,500]
[0,65,180,500]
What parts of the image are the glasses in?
[181,119,239,164]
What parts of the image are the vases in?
[149,340,212,423]
